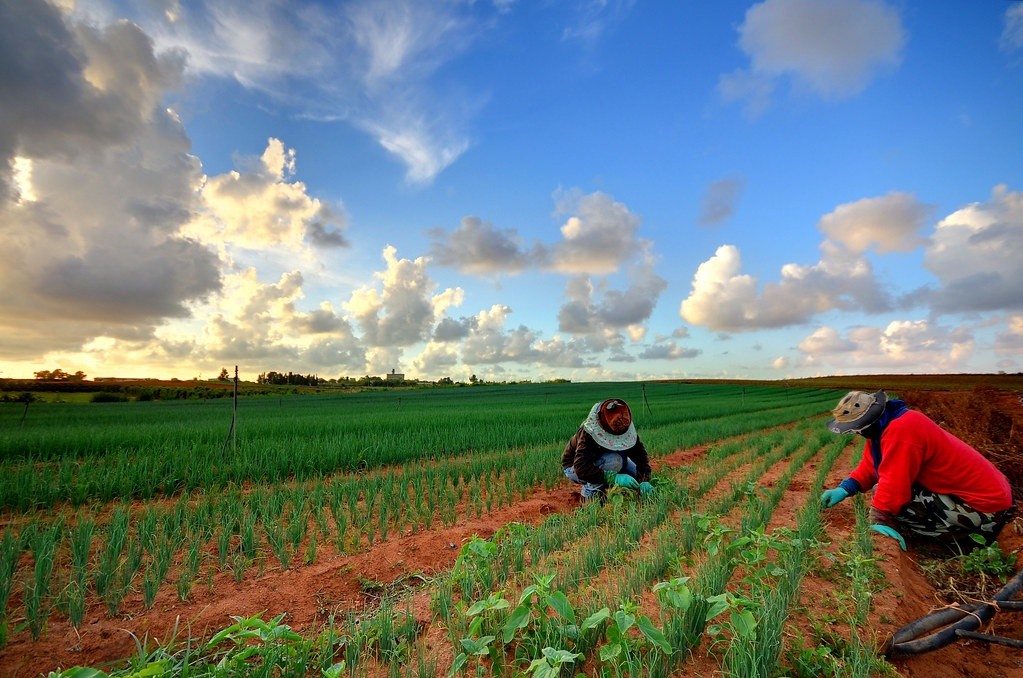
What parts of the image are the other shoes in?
[945,536,971,558]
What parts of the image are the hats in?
[827,389,885,433]
[584,398,638,451]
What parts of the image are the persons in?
[820,389,1014,560]
[561,398,654,503]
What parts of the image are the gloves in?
[821,486,850,508]
[639,481,655,497]
[870,524,907,552]
[615,473,640,489]
